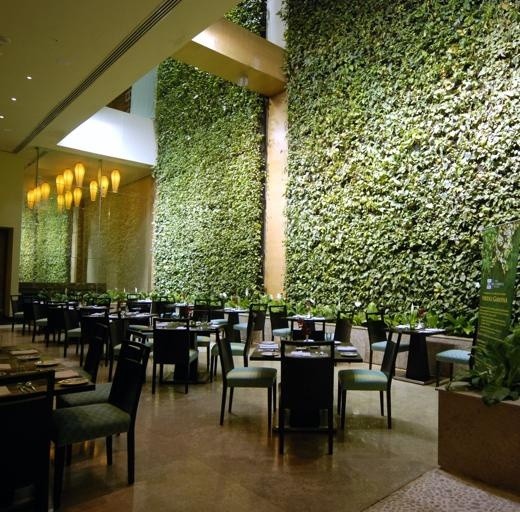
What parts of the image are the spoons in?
[26,381,39,392]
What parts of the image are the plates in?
[17,354,41,359]
[34,360,58,365]
[261,340,275,344]
[335,340,341,344]
[260,351,279,356]
[341,352,358,357]
[56,377,89,386]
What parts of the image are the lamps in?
[237,71,248,87]
[89,160,120,202]
[56,163,85,213]
[26,149,51,210]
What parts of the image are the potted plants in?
[435,326,520,491]
